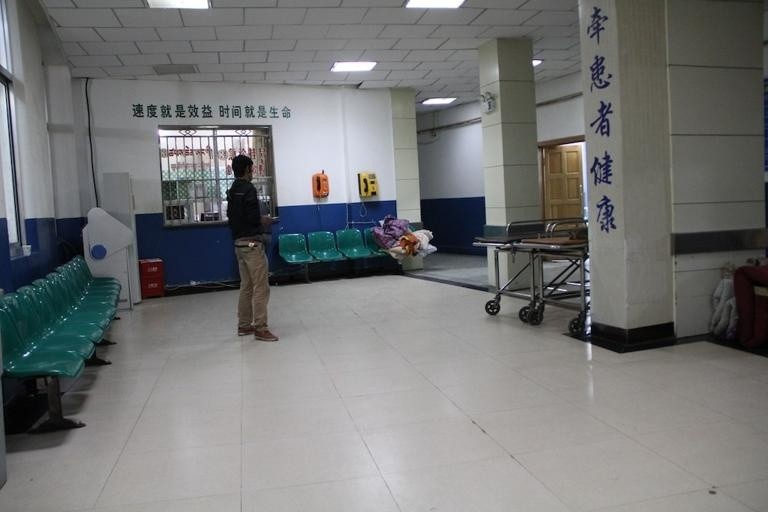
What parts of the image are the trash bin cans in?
[139,258,165,299]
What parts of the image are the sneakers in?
[236,325,255,335]
[253,329,279,342]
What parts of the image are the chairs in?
[279,223,413,284]
[0,255,121,435]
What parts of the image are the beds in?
[471,215,589,337]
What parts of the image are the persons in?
[225,155,279,342]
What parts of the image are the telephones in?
[358,173,378,197]
[312,170,330,198]
